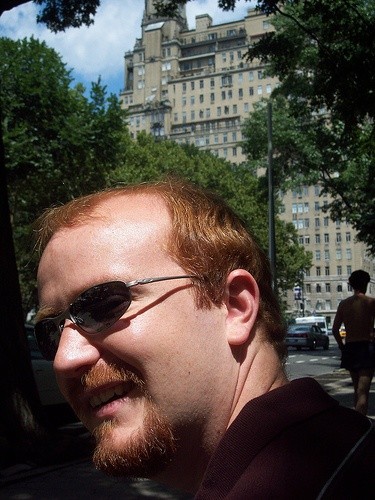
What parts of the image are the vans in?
[294,317,328,336]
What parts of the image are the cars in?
[283,324,329,352]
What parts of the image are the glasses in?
[33,273,207,361]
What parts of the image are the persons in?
[30,178,375,500]
[331,270,375,416]
